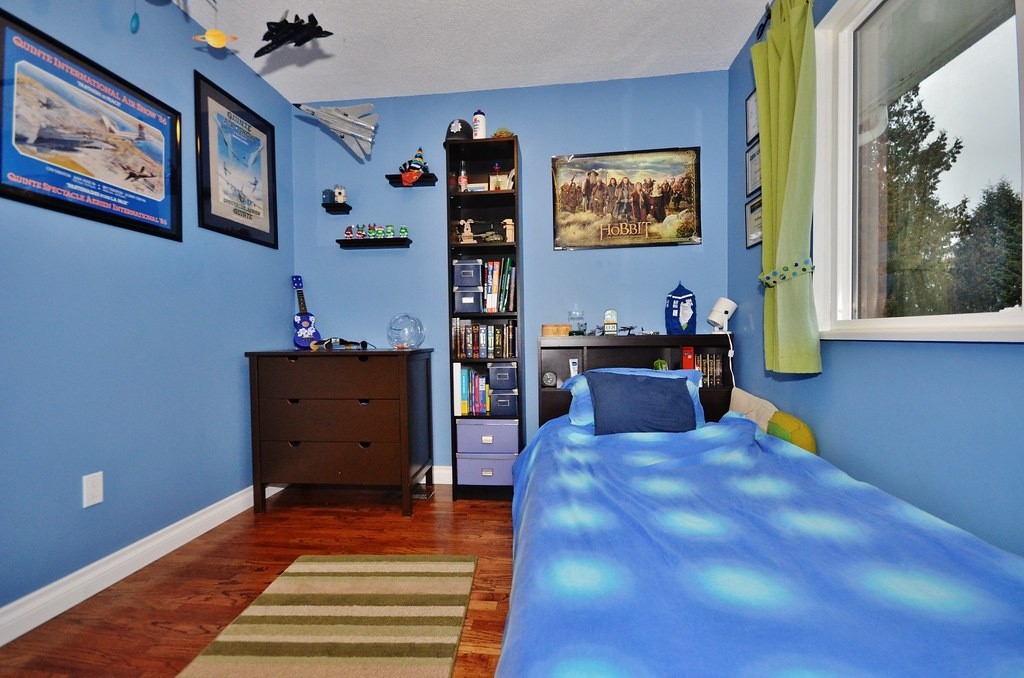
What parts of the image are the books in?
[682,346,723,388]
[453,362,491,416]
[483,257,515,313]
[452,318,518,360]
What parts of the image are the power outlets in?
[82,470,104,509]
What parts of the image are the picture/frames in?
[550,145,702,251]
[0,6,183,243]
[193,68,279,251]
[744,88,764,250]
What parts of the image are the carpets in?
[174,552,479,678]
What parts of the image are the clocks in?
[542,370,557,388]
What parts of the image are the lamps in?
[706,298,738,334]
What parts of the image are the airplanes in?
[291,102,379,164]
[253,9,335,59]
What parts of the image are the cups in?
[568,311,584,336]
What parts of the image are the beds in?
[491,414,1024,678]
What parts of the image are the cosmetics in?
[569,359,579,377]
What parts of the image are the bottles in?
[386,312,426,348]
[472,108,487,139]
[458,161,468,192]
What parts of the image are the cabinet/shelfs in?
[245,347,435,513]
[538,333,734,424]
[444,136,527,502]
[320,173,438,249]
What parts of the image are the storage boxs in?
[455,419,519,486]
[452,258,484,314]
[486,362,519,417]
[488,174,509,191]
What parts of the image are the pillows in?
[561,368,817,456]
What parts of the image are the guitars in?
[291,275,321,350]
